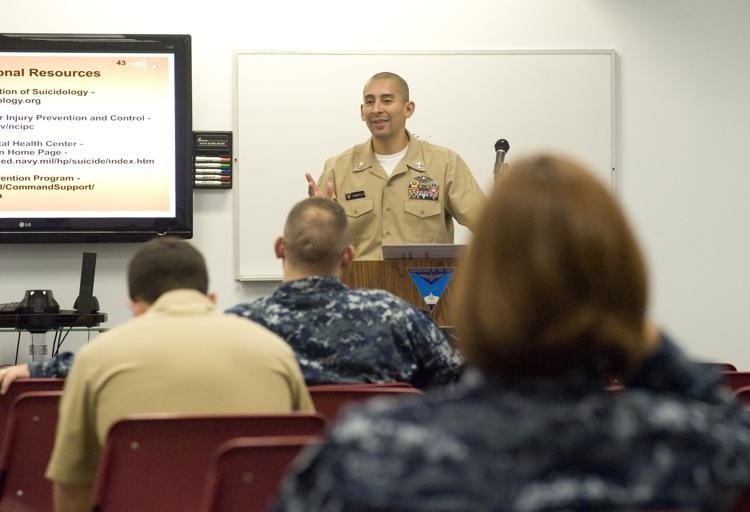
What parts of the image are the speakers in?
[73,252,100,313]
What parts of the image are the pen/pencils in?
[195,156,232,189]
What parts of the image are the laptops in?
[382,244,467,260]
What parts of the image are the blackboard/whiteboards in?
[233,49,617,283]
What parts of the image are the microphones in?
[493,138,510,177]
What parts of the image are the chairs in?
[0,377,425,512]
[718,363,750,409]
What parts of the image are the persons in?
[305,71,488,261]
[46,238,324,511]
[263,153,748,511]
[1,195,470,399]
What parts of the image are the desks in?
[0,328,111,369]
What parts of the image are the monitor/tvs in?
[0,33,193,244]
